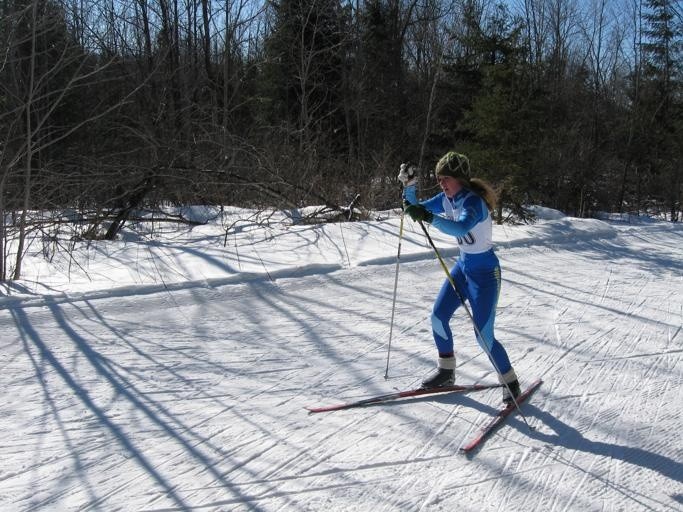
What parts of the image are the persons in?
[397,151,520,406]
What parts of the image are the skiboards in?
[302,378,543,451]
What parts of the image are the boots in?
[420,356,455,387]
[497,367,519,404]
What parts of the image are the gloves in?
[403,204,433,225]
[397,163,419,186]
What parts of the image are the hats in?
[434,151,471,187]
